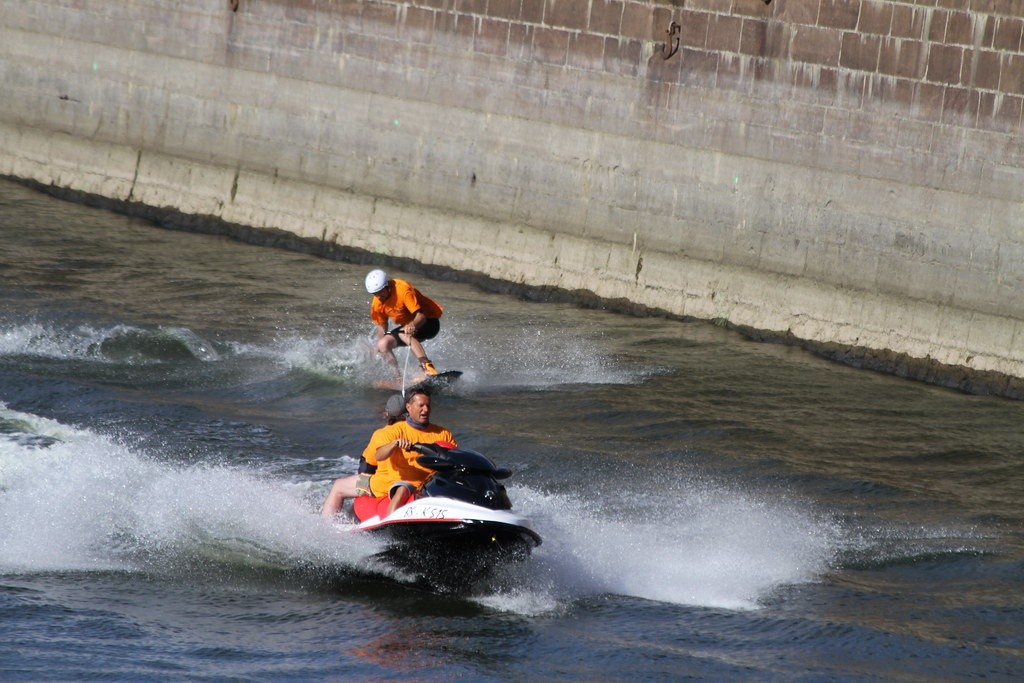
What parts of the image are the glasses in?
[406,389,431,403]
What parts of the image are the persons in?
[324,394,406,512]
[374,390,459,516]
[364,270,443,388]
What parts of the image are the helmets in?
[364,269,389,293]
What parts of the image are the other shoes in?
[368,375,403,389]
[412,360,440,383]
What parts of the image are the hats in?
[385,395,406,416]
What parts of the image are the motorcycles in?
[337,439,544,565]
